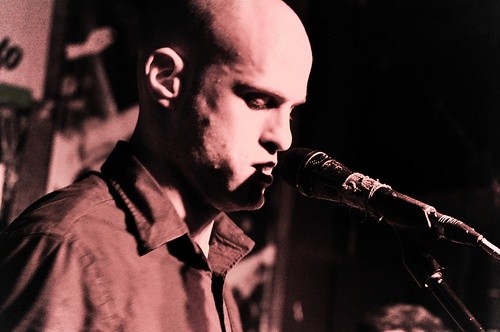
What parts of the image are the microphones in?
[278,148,483,248]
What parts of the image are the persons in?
[0,0,313,332]
